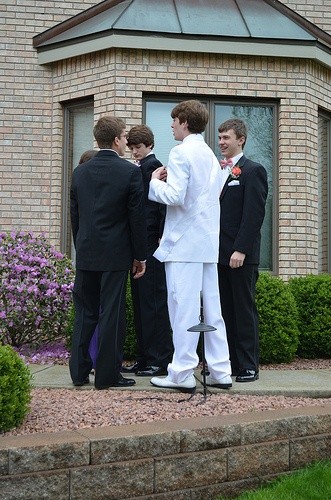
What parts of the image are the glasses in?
[120,134,130,140]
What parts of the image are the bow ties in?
[220,158,233,168]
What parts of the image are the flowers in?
[135,163,141,166]
[228,166,242,179]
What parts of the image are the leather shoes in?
[235,368,259,382]
[108,374,136,387]
[120,362,141,373]
[150,376,196,394]
[135,366,168,376]
[72,375,89,386]
[200,369,210,375]
[202,375,232,388]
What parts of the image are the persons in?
[148,100,233,394]
[121,125,175,376]
[200,119,269,382]
[69,117,148,390]
